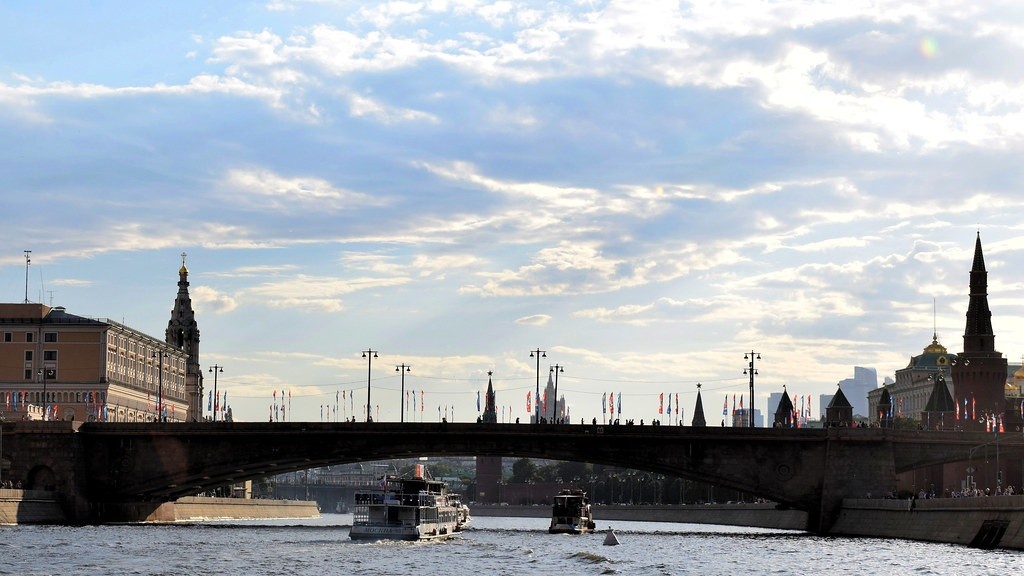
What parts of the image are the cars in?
[881,490,913,499]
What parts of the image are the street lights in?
[530,348,547,424]
[209,364,224,422]
[362,348,378,422]
[38,366,53,420]
[952,356,970,432]
[152,349,168,422]
[927,374,944,431]
[978,410,1005,495]
[396,363,410,423]
[550,364,564,424]
[744,350,761,427]
[743,362,758,427]
[471,472,665,505]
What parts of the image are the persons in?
[0,479,21,489]
[540,416,564,424]
[351,416,355,422]
[346,417,349,422]
[476,415,483,423]
[581,417,725,427]
[442,418,447,423]
[867,485,1017,499]
[516,417,520,423]
[772,421,960,430]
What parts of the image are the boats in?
[348,474,463,542]
[447,493,470,523]
[549,489,597,534]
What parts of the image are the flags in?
[722,394,743,415]
[495,406,511,423]
[527,390,547,413]
[46,405,58,421]
[477,391,496,411]
[406,390,424,411]
[4,390,25,410]
[791,391,1024,432]
[320,390,353,422]
[364,404,379,422]
[602,392,621,413]
[208,390,227,411]
[85,392,107,423]
[269,390,291,422]
[659,393,678,414]
[438,406,453,422]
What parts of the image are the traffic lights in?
[998,472,1002,483]
